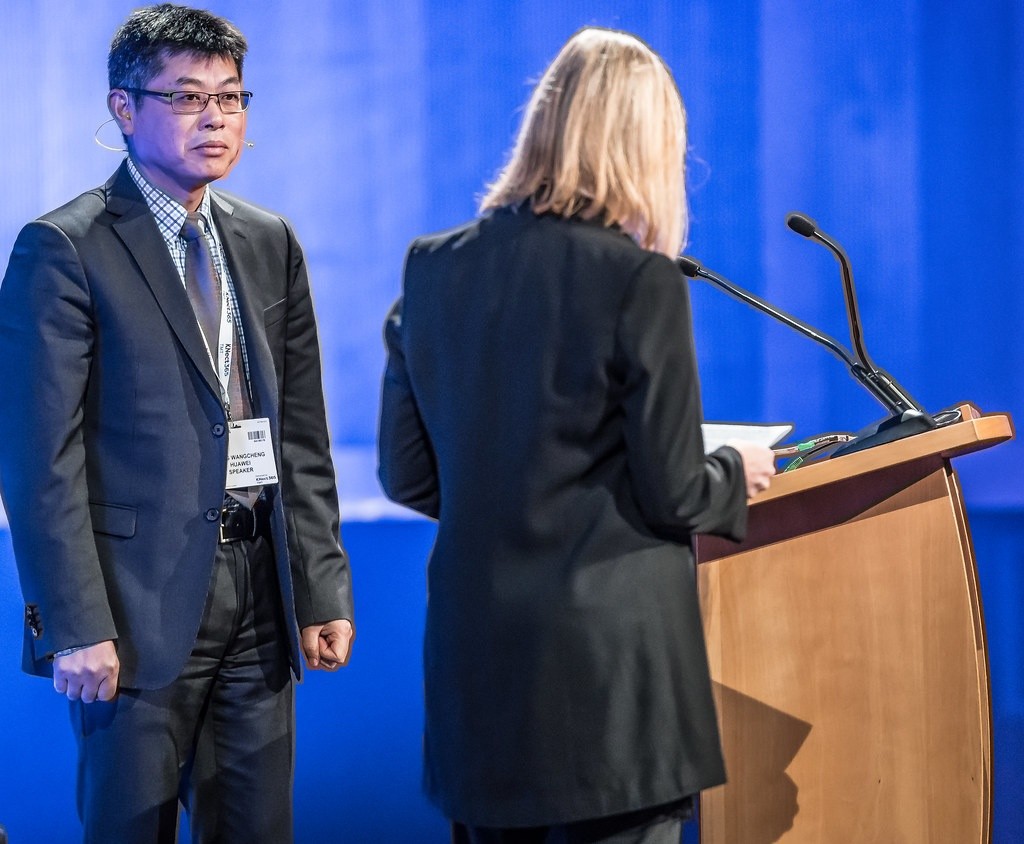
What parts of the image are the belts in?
[216,501,277,542]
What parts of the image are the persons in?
[378,29,776,844]
[1,5,354,843]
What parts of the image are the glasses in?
[117,84,255,115]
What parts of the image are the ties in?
[181,212,264,511]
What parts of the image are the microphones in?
[242,140,254,148]
[676,214,937,459]
